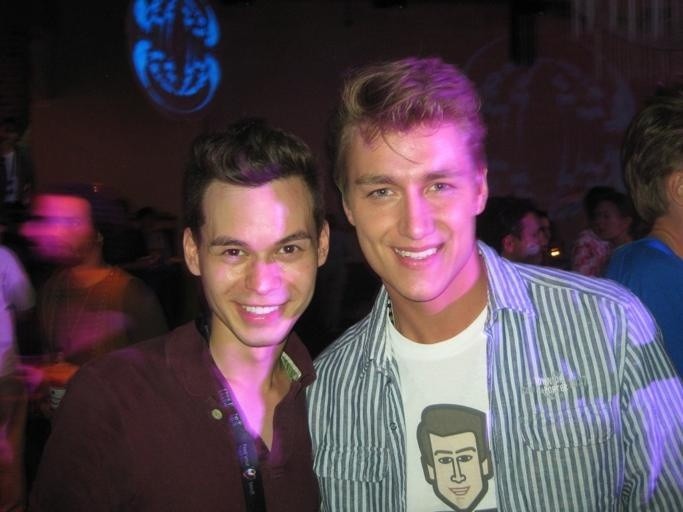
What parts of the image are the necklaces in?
[66,273,100,339]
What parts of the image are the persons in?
[479,185,641,275]
[0,98,32,208]
[303,52,682,512]
[24,118,325,510]
[1,184,214,512]
[595,80,683,380]
